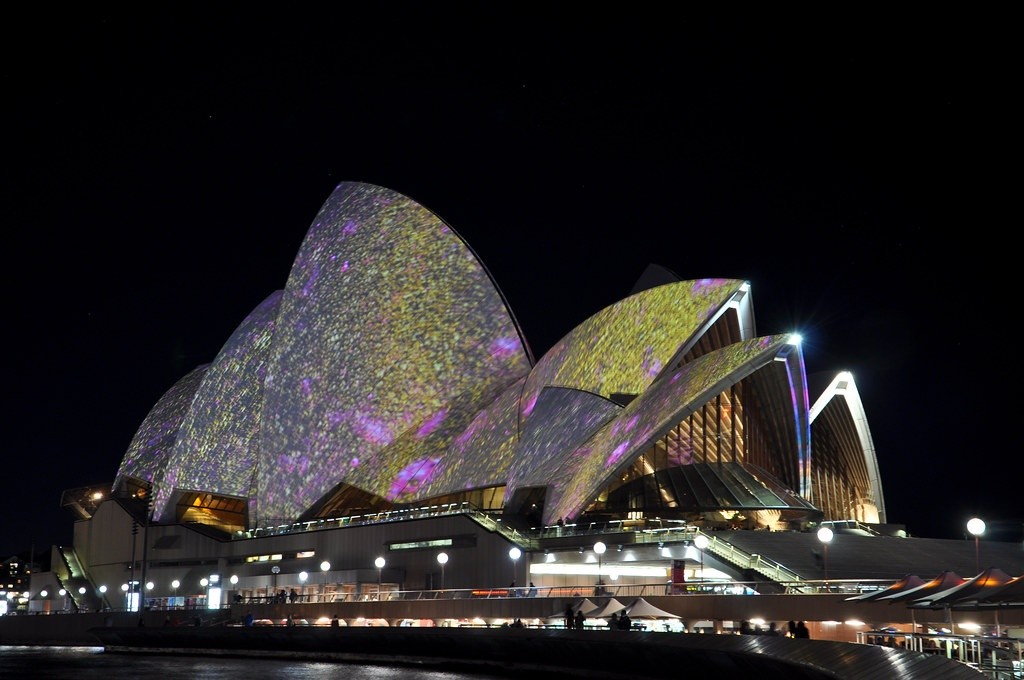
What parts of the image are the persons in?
[331,614,340,626]
[285,614,295,626]
[278,589,298,603]
[868,635,994,660]
[607,610,631,631]
[508,580,537,597]
[243,610,254,627]
[740,620,809,640]
[137,612,203,628]
[564,605,587,631]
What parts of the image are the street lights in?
[299,571,308,601]
[172,579,180,608]
[200,578,209,610]
[967,518,986,575]
[509,547,521,597]
[817,527,834,592]
[271,565,280,598]
[320,560,331,603]
[376,556,386,599]
[694,534,707,594]
[230,575,238,591]
[593,542,607,594]
[59,589,66,613]
[121,584,129,611]
[99,586,107,612]
[79,587,86,614]
[40,590,47,614]
[437,552,449,599]
